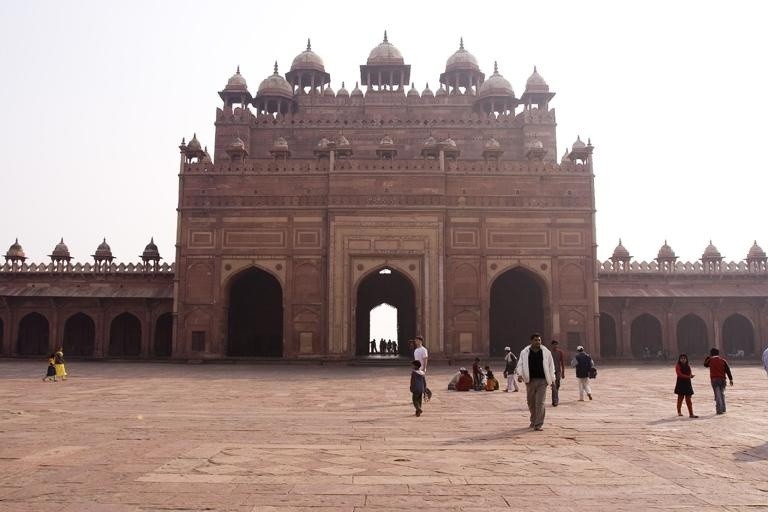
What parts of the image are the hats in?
[505,347,510,351]
[576,345,584,351]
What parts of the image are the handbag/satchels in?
[586,355,597,379]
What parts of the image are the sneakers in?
[577,400,585,401]
[530,423,543,431]
[417,409,423,417]
[678,414,683,416]
[588,394,593,400]
[716,409,726,414]
[42,377,68,383]
[689,415,699,418]
[502,389,518,392]
[552,401,558,407]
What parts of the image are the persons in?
[548,340,565,406]
[448,356,499,392]
[501,347,518,393]
[517,333,556,431]
[571,345,594,402]
[726,344,754,360]
[42,346,67,383]
[641,338,667,361]
[704,348,733,415]
[674,354,699,418]
[368,339,400,357]
[410,335,428,418]
[762,345,768,375]
